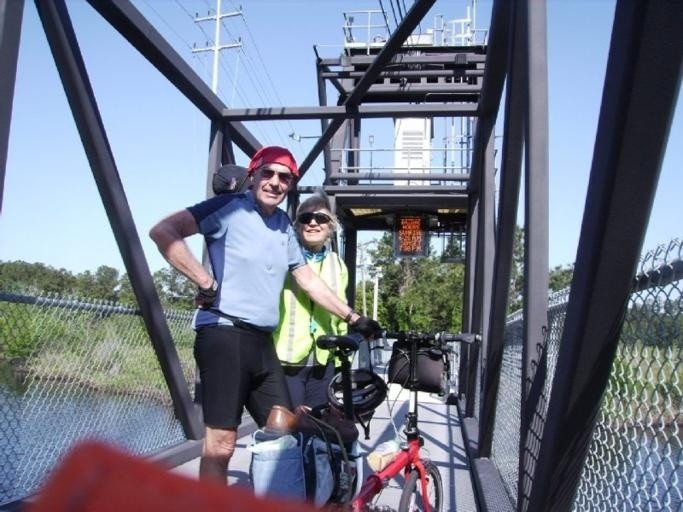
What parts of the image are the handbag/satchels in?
[247,430,343,507]
[391,342,447,392]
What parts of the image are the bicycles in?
[272,320,485,512]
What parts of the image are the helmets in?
[327,369,386,422]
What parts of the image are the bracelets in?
[344,309,356,323]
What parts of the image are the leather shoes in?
[266,405,358,441]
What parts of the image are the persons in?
[270,196,349,416]
[149,146,382,491]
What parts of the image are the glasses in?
[259,169,291,184]
[299,212,330,224]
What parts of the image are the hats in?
[249,146,299,178]
